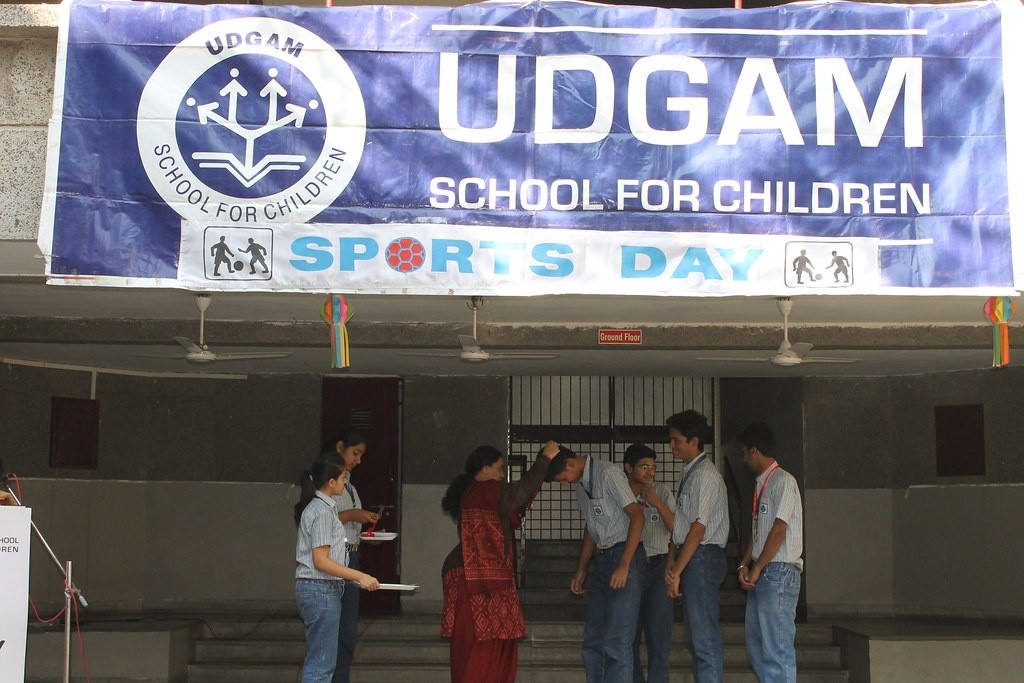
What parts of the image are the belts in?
[345,542,359,551]
[647,555,667,564]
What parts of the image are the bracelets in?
[737,565,744,572]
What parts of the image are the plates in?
[359,531,398,540]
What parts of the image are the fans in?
[398,297,561,363]
[696,298,863,368]
[129,295,292,363]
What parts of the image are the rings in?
[373,517,375,519]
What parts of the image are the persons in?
[536,445,648,683]
[738,424,804,683]
[664,409,729,683]
[622,446,677,683]
[295,430,380,683]
[442,441,561,683]
